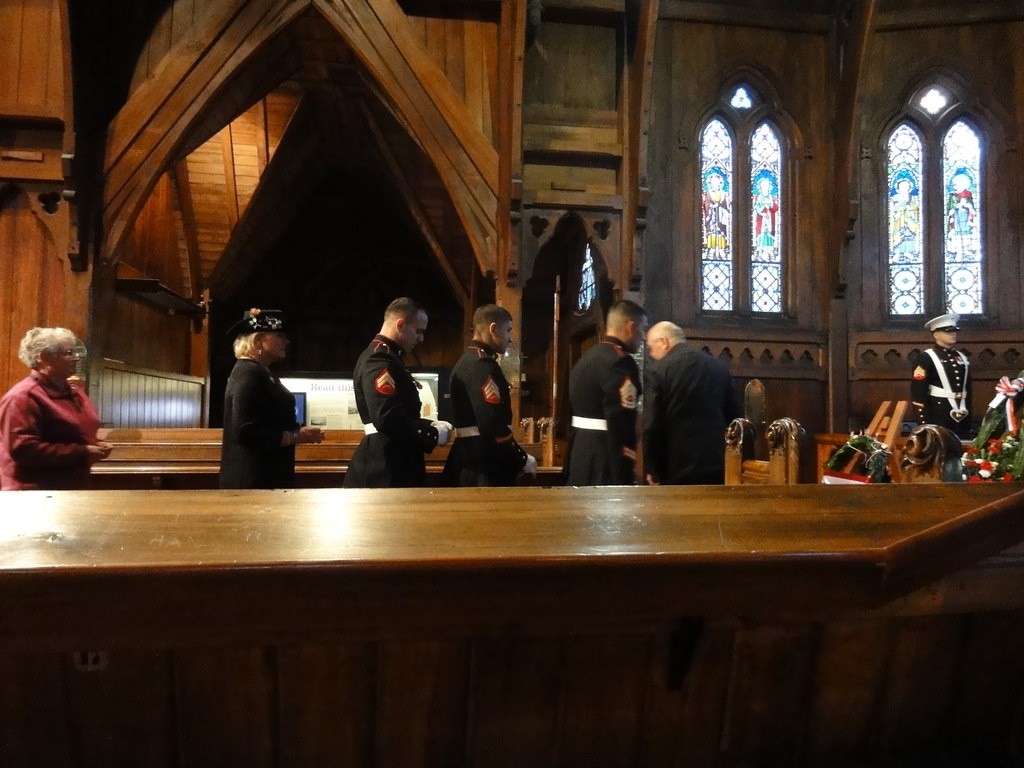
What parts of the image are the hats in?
[924,313,960,332]
[227,308,284,341]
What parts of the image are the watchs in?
[293,430,302,444]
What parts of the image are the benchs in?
[24,427,594,489]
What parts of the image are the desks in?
[0,482,1024,768]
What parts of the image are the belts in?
[571,415,608,430]
[364,422,377,436]
[452,424,513,439]
[928,384,967,400]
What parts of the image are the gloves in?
[522,454,537,473]
[430,420,453,447]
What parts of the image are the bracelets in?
[286,431,291,447]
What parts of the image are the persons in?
[640,321,739,485]
[441,303,538,488]
[340,296,452,488]
[560,300,649,486]
[218,309,326,488]
[910,313,975,439]
[0,326,115,491]
